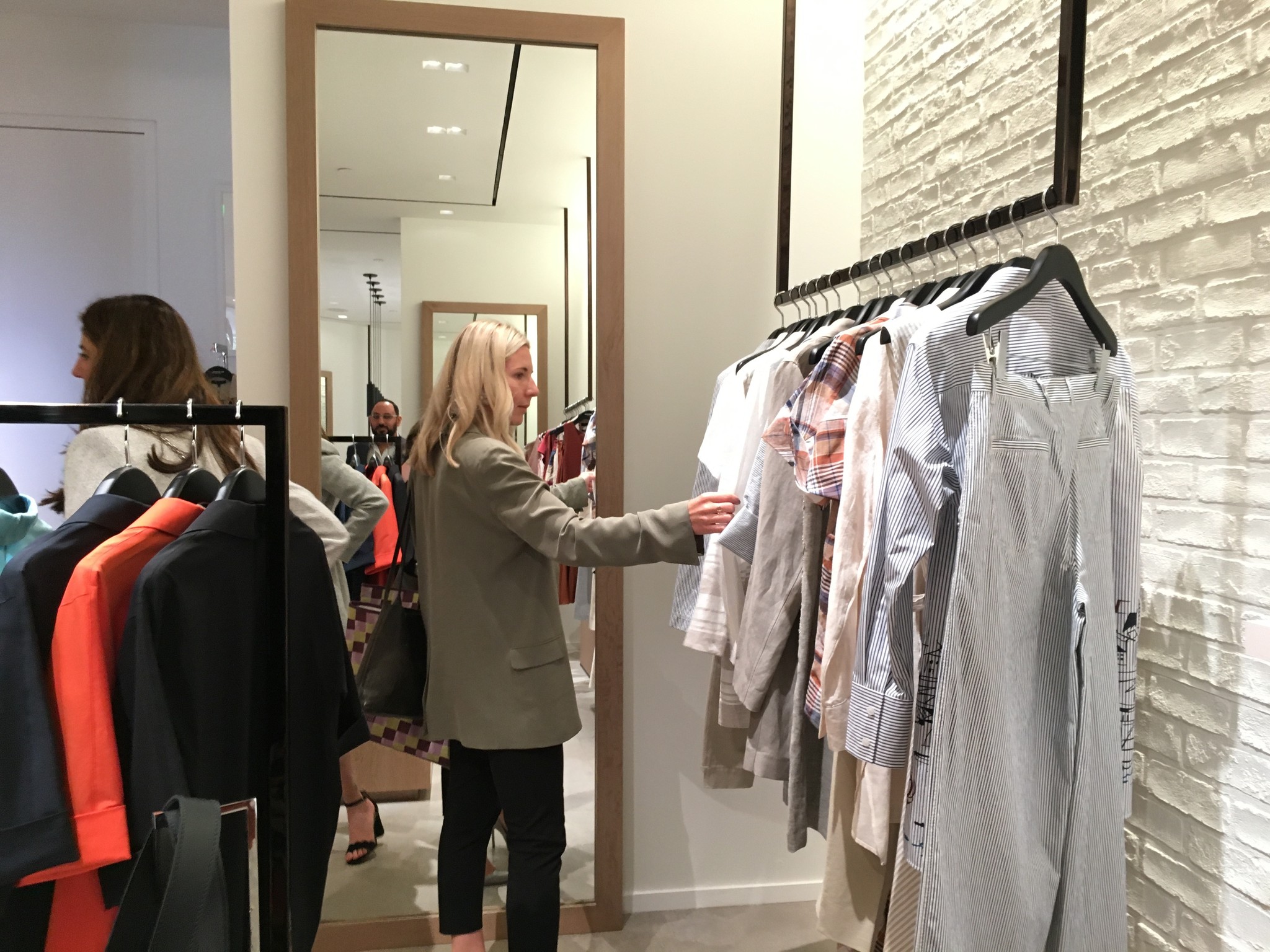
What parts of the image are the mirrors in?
[283,0,625,952]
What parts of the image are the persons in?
[408,421,595,885]
[347,398,406,481]
[37,293,264,522]
[408,321,741,952]
[289,437,385,865]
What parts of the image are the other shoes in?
[483,869,511,886]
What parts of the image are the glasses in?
[370,414,398,420]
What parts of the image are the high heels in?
[490,817,512,848]
[343,790,385,866]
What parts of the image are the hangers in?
[735,177,1116,377]
[537,396,594,440]
[91,396,268,506]
[348,433,395,476]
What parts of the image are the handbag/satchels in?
[341,483,461,766]
